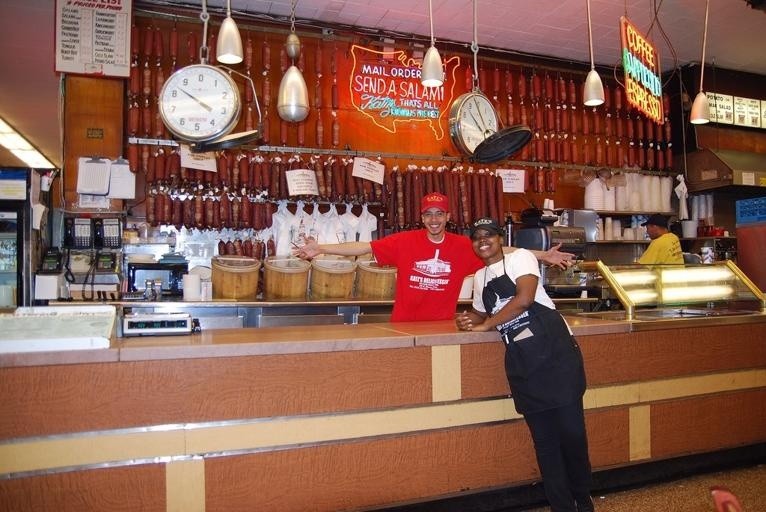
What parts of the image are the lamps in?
[690,0,710,125]
[421,0,444,87]
[583,0,605,107]
[216,0,245,66]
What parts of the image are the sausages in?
[130,143,558,233]
[217,237,277,261]
[127,27,341,149]
[465,65,673,170]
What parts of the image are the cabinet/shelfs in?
[586,210,693,245]
[686,236,737,264]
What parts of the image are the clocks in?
[448,0,499,158]
[158,0,242,144]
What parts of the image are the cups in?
[692,194,714,226]
[598,217,644,240]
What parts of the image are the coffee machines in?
[515,228,588,300]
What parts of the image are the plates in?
[126,251,185,265]
[583,174,672,213]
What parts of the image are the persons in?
[635,211,689,309]
[291,191,577,322]
[455,216,597,511]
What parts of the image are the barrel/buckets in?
[212,255,261,302]
[263,256,311,301]
[355,260,398,300]
[311,256,356,302]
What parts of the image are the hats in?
[420,192,450,214]
[469,216,504,239]
[641,213,668,228]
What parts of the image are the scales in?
[122,312,193,337]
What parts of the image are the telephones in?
[94,217,121,250]
[63,216,94,249]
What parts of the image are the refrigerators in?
[0,166,48,312]
